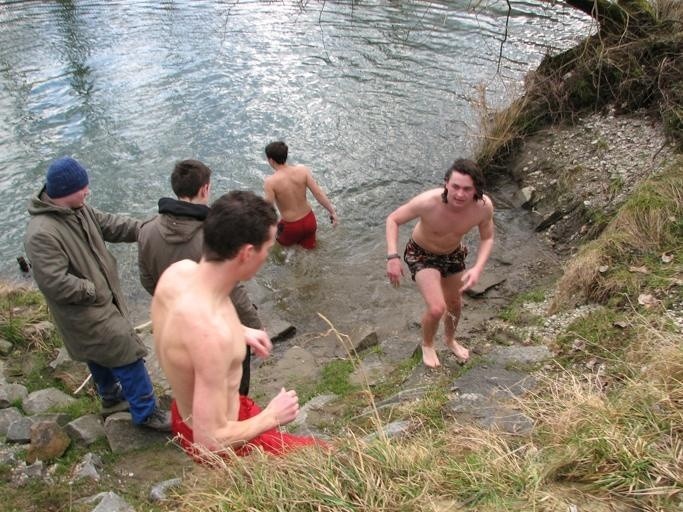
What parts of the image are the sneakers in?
[101,384,124,407]
[141,407,172,432]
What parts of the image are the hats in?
[46,159,89,198]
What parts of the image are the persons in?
[149,189,331,470]
[384,156,494,368]
[22,156,173,433]
[262,141,338,251]
[137,159,266,397]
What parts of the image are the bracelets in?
[386,253,399,260]
